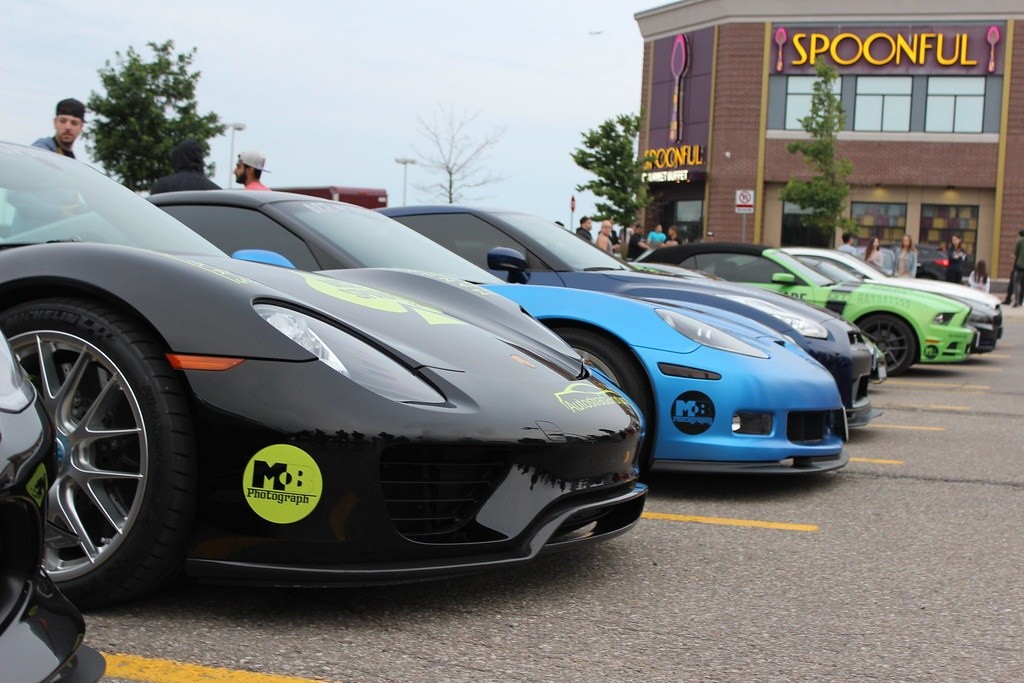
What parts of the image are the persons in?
[150,140,222,195]
[596,220,613,254]
[892,235,917,277]
[598,216,620,253]
[946,233,968,282]
[863,237,883,269]
[646,224,666,247]
[626,225,650,260]
[967,260,991,293]
[576,216,592,241]
[838,233,857,256]
[1001,230,1024,308]
[233,150,274,191]
[662,225,683,247]
[6,97,87,228]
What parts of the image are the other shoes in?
[1013,302,1019,308]
[1001,298,1011,305]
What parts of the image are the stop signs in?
[571,198,575,214]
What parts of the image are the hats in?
[580,216,589,225]
[239,149,271,173]
[57,98,86,123]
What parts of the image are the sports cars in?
[0,138,651,616]
[625,237,1005,376]
[362,203,890,429]
[0,189,852,489]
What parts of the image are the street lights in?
[396,157,417,203]
